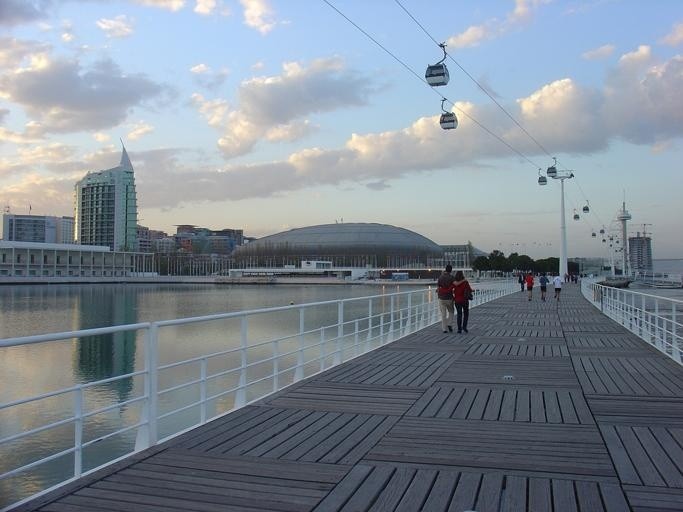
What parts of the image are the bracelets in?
[462,279,465,282]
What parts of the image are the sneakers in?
[448,325,453,332]
[462,327,469,333]
[443,330,448,333]
[457,329,462,334]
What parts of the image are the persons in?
[539,273,549,301]
[564,272,587,284]
[518,272,526,291]
[524,272,534,301]
[552,273,562,301]
[435,270,472,334]
[437,264,467,333]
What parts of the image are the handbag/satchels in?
[463,281,473,301]
